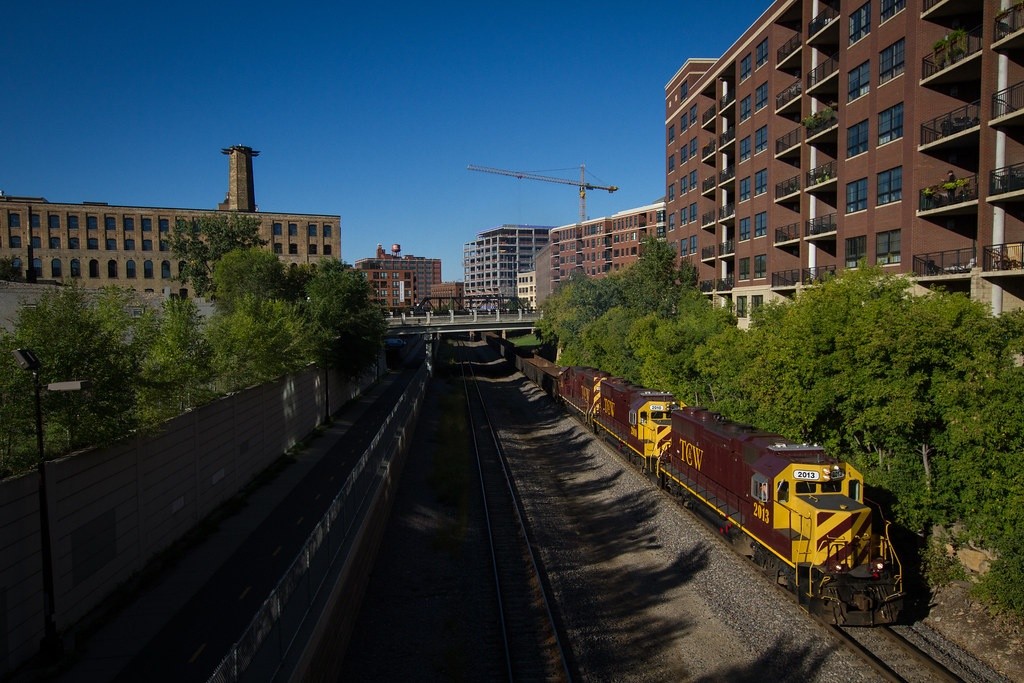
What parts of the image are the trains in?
[478,330,906,630]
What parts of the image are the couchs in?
[1001,174,1024,192]
[925,259,939,276]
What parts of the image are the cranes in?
[466,162,620,223]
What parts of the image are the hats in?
[946,170,953,174]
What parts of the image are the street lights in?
[16,348,84,666]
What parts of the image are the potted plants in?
[934,54,946,73]
[948,47,963,64]
[921,178,969,199]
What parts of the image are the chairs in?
[944,258,976,273]
[940,116,980,137]
